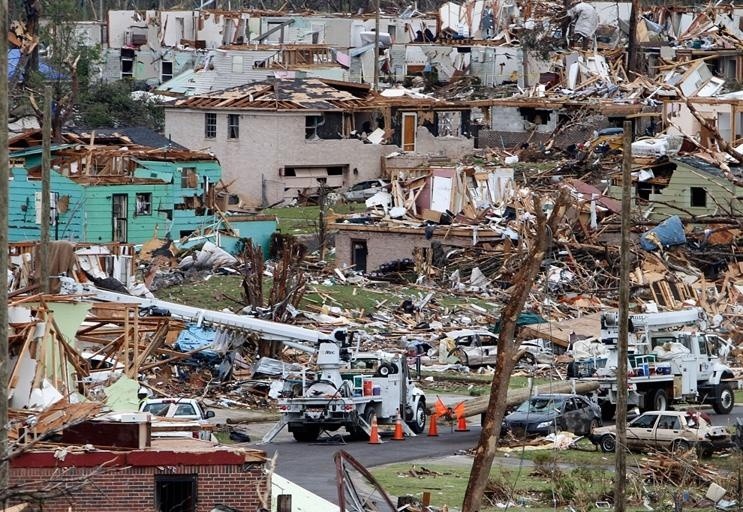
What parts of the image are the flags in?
[453,400,465,419]
[433,398,447,418]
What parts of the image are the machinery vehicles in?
[567,307,740,420]
[278,347,426,442]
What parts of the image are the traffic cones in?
[456,416,470,432]
[392,412,404,440]
[427,415,438,436]
[368,413,382,445]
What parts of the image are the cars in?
[138,397,214,440]
[590,411,735,455]
[500,393,602,437]
